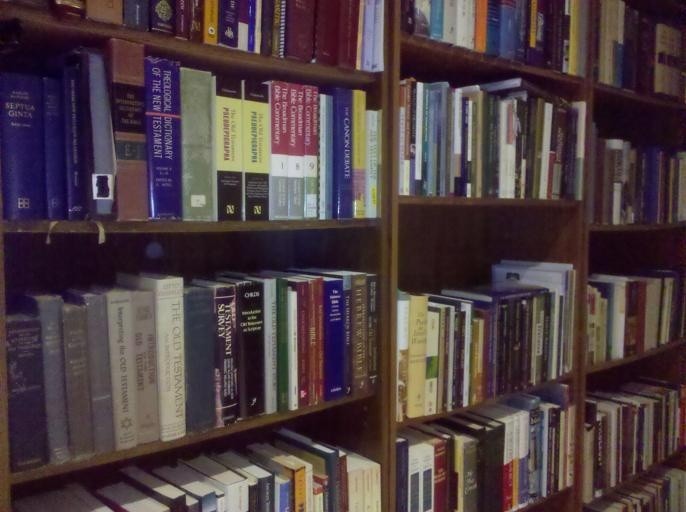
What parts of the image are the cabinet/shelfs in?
[392,0,593,512]
[0,0,386,512]
[578,0,686,512]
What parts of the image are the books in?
[393,0,686,226]
[3,2,380,220]
[393,254,686,512]
[1,272,386,512]
